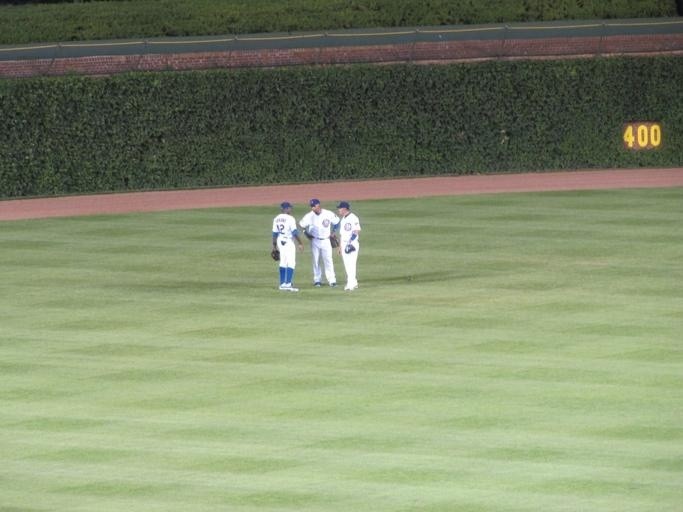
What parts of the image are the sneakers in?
[279,281,360,293]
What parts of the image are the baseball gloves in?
[331,236,338,248]
[271,251,279,260]
[344,244,355,253]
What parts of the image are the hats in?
[310,198,320,206]
[336,201,350,209]
[280,202,293,209]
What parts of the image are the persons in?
[296,198,341,288]
[272,202,305,292]
[336,201,361,291]
